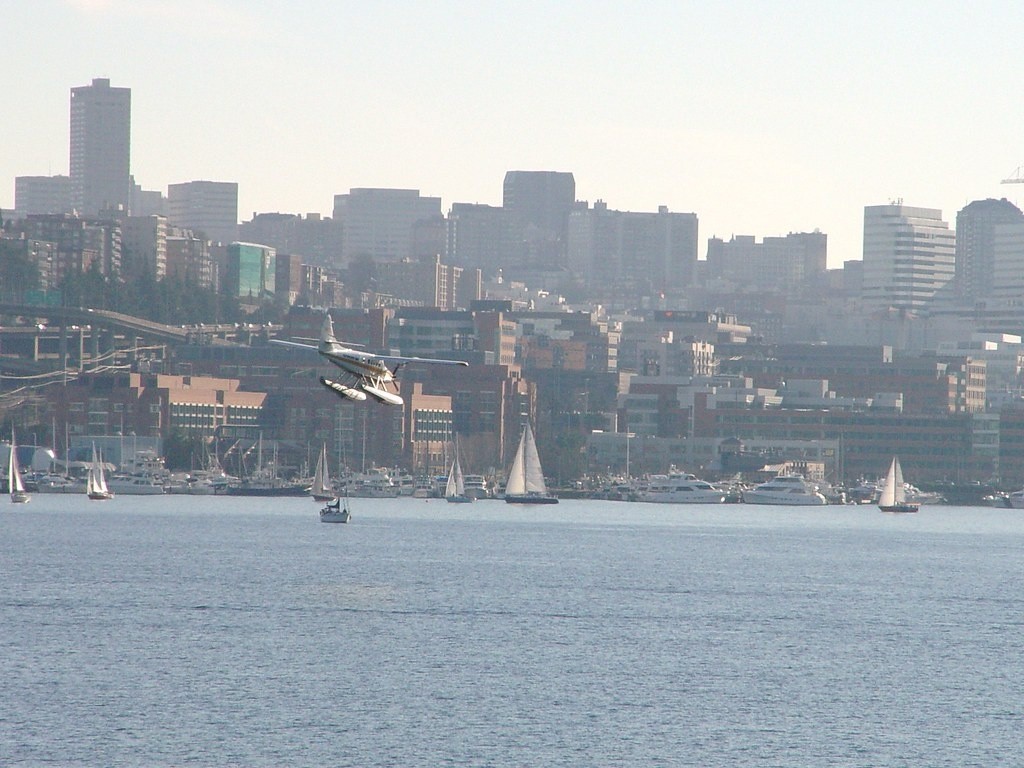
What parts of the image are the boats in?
[847,477,943,508]
[741,472,827,505]
[990,497,1012,507]
[618,472,731,504]
[1010,489,1024,509]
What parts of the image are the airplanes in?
[267,314,469,405]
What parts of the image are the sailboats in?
[319,411,352,523]
[878,456,922,513]
[7,421,29,503]
[0,412,558,506]
